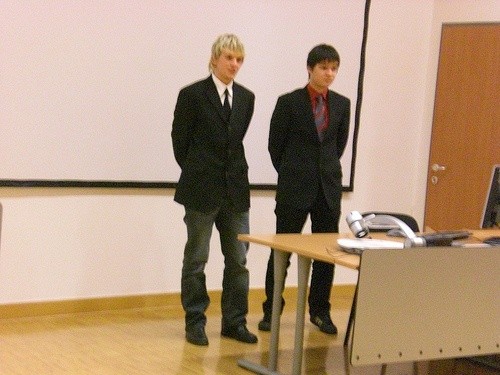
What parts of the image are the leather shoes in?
[186,327,209,345]
[221,325,258,343]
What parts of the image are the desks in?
[236,230,500,375]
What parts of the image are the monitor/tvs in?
[480,164,500,229]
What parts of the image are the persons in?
[171,33,259,347]
[257,45,350,335]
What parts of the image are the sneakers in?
[310,313,337,334]
[259,318,271,331]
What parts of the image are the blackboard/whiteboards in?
[0,0,370,191]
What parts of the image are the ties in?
[314,96,325,139]
[223,90,233,120]
